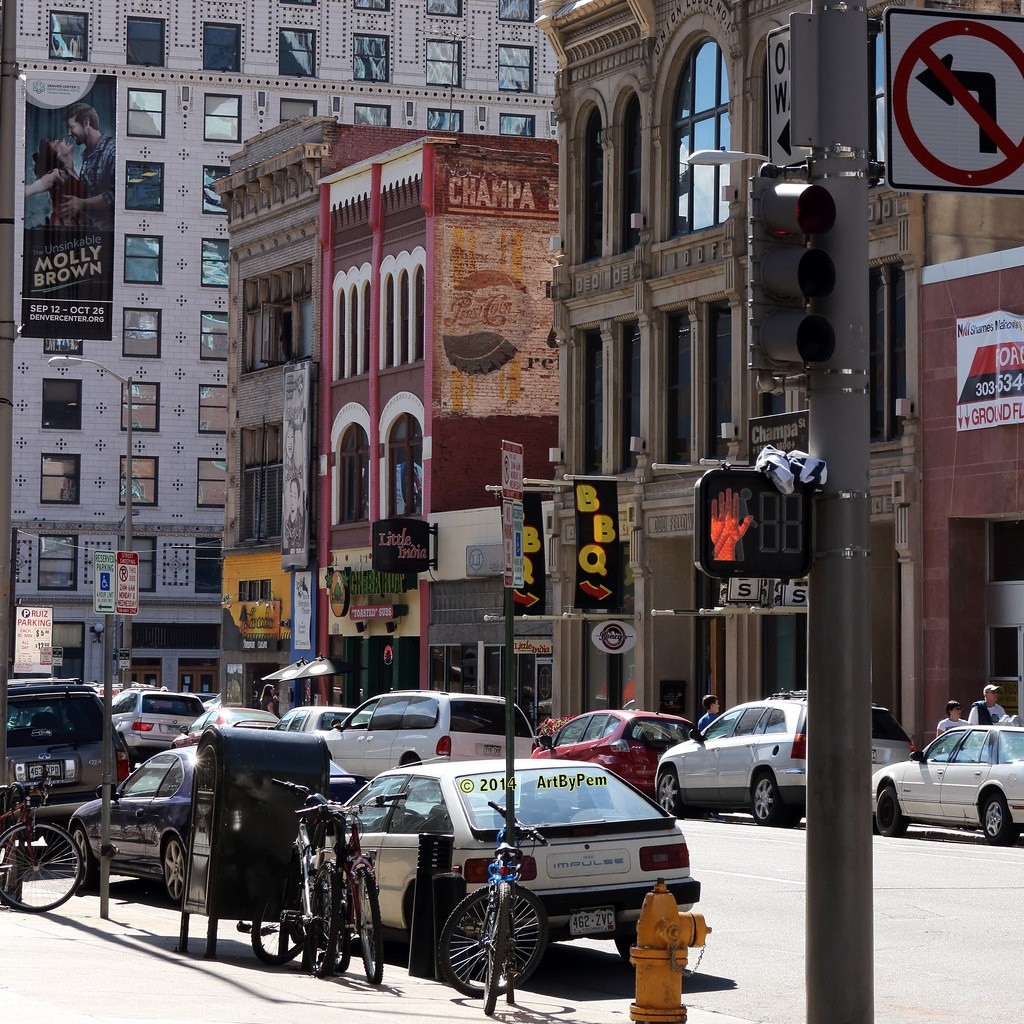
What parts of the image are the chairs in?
[430,796,560,828]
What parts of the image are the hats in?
[983,684,1001,696]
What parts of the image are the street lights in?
[48,353,134,691]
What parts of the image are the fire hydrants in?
[627,876,711,1024]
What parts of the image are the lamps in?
[90,623,104,643]
[549,448,564,462]
[721,422,738,439]
[221,592,274,607]
[630,213,646,228]
[722,186,738,201]
[549,236,564,250]
[385,622,397,633]
[630,436,646,452]
[356,621,367,633]
[895,398,914,416]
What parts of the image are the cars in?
[872,725,1024,847]
[310,759,702,970]
[67,746,356,906]
[528,709,698,802]
[87,680,280,772]
[267,705,374,735]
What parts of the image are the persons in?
[697,694,721,732]
[258,683,282,720]
[936,699,971,754]
[968,684,1006,725]
[151,686,172,709]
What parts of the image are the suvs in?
[325,689,538,782]
[5,678,129,851]
[655,689,916,829]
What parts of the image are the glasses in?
[956,708,962,710]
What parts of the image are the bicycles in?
[251,776,408,985]
[0,763,85,913]
[440,799,551,1015]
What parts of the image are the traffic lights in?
[748,174,839,377]
[697,468,814,580]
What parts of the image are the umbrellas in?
[260,658,310,683]
[279,655,370,705]
